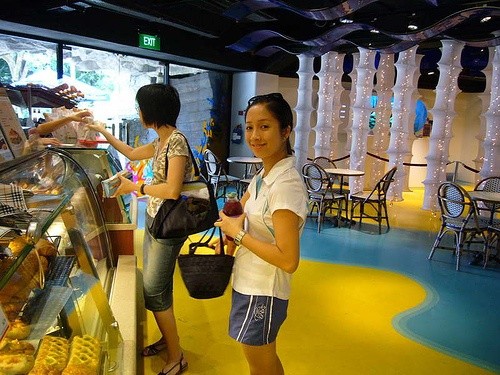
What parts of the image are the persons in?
[209,93,309,375]
[23,111,92,145]
[86,83,194,375]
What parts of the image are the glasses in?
[248,92,283,106]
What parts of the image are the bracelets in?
[140,184,146,195]
[234,229,246,246]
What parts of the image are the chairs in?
[301,157,350,234]
[427,180,500,271]
[202,149,253,202]
[350,165,397,235]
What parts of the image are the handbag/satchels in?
[178,227,235,299]
[149,179,220,240]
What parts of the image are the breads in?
[0,235,55,322]
[21,182,64,195]
[0,320,102,375]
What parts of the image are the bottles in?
[222,192,244,241]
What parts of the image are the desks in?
[227,157,263,199]
[465,191,500,265]
[322,168,366,227]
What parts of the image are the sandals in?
[158,353,188,375]
[145,337,165,355]
